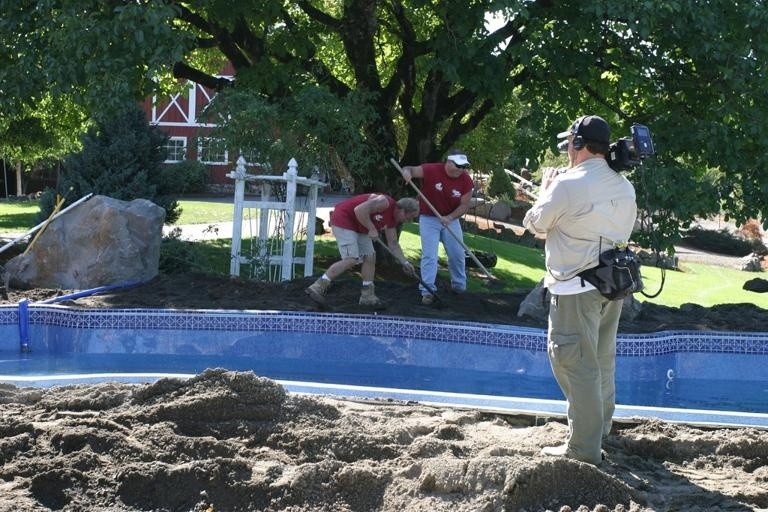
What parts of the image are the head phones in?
[573,116,589,150]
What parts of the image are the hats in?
[557,115,610,145]
[446,153,470,167]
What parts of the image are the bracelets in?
[448,214,453,221]
[404,261,409,264]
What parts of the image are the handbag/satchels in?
[576,244,644,302]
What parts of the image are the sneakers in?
[541,439,604,469]
[447,282,469,304]
[421,292,438,305]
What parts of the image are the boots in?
[358,283,388,310]
[305,276,332,309]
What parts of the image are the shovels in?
[4,186,74,292]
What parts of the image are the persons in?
[523,114,637,465]
[304,192,420,309]
[403,148,474,305]
